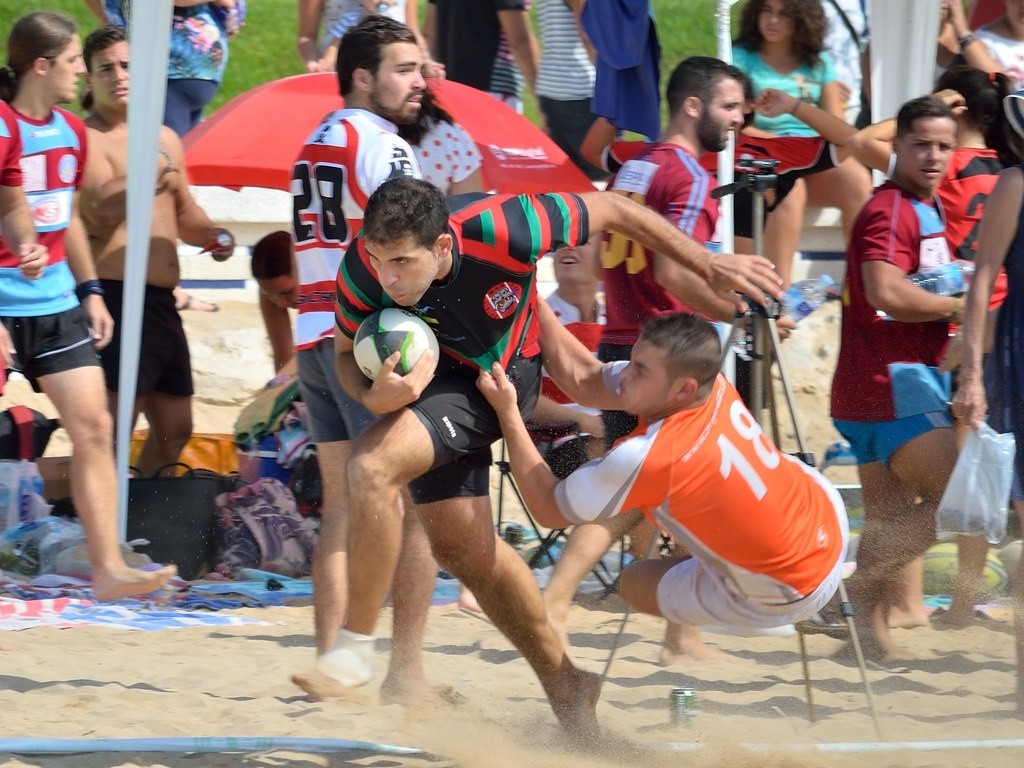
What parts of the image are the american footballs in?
[921,541,1009,606]
[352,307,440,381]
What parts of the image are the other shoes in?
[846,638,909,673]
[796,610,851,640]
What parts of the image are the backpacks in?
[215,478,318,579]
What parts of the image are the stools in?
[495,434,671,599]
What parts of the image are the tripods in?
[605,159,883,745]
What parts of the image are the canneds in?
[669,688,698,729]
[504,524,525,550]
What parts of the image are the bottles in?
[876,259,975,321]
[0,462,44,535]
[733,274,834,362]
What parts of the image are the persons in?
[0,98,50,399]
[76,25,235,477]
[516,56,753,666]
[252,230,297,374]
[83,0,244,141]
[296,0,1024,326]
[523,229,692,562]
[477,292,849,628]
[289,16,485,676]
[292,176,785,757]
[830,96,967,662]
[952,85,1024,717]
[0,12,177,604]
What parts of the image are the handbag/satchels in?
[128,463,221,581]
[0,460,150,576]
[934,421,1014,545]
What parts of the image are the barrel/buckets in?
[123,463,251,579]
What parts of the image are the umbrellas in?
[180,71,605,197]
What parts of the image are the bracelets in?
[951,297,958,320]
[73,278,103,306]
[159,149,174,173]
[791,99,801,116]
